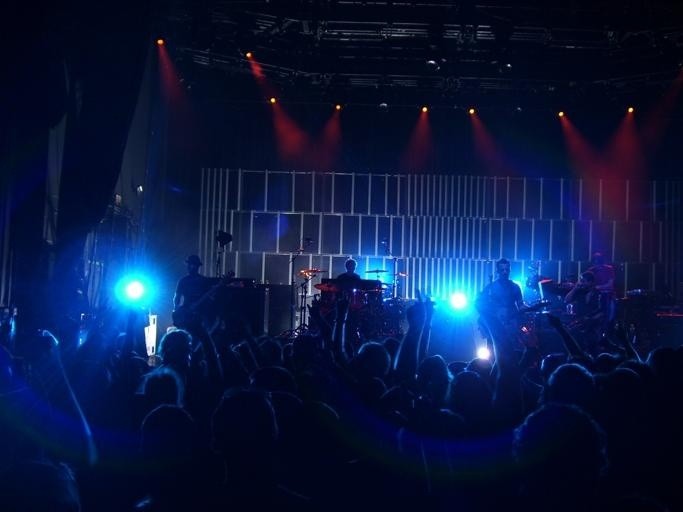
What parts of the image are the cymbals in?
[317,281,337,292]
[367,269,388,273]
[299,268,326,276]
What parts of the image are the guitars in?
[477,301,551,337]
[173,271,235,329]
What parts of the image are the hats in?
[186,255,203,265]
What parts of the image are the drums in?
[347,289,368,312]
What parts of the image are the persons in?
[1,256,683,512]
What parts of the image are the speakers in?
[651,305,683,349]
[263,283,297,336]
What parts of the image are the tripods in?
[294,286,308,334]
